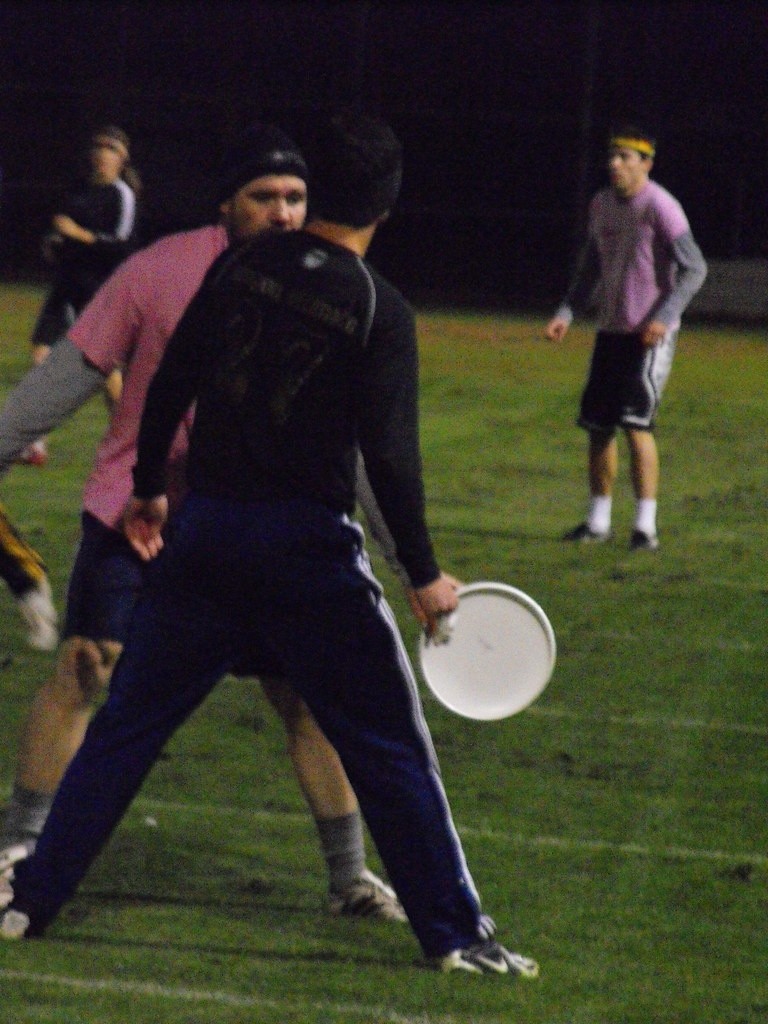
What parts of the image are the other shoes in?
[16,581,61,652]
[1,845,30,904]
[630,524,660,553]
[328,870,408,922]
[16,444,51,466]
[560,524,614,544]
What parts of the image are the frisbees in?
[416,577,561,723]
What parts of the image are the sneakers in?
[443,918,541,980]
[1,909,30,942]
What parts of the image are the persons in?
[0,132,427,921]
[0,500,59,649]
[0,112,541,979]
[7,125,142,464]
[544,126,707,551]
